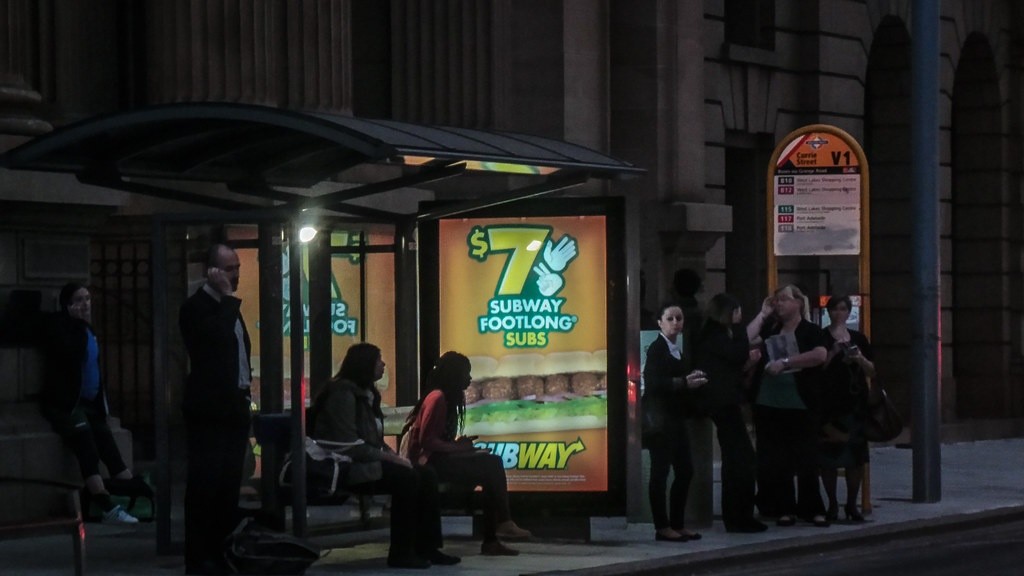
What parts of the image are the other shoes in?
[482,542,519,555]
[812,515,826,526]
[416,548,460,564]
[777,513,797,526]
[724,518,768,532]
[184,560,229,576]
[388,545,431,568]
[656,530,701,541]
[495,520,532,538]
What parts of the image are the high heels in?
[844,502,864,522]
[825,506,839,520]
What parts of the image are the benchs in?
[240,495,391,545]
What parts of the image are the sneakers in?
[101,505,139,526]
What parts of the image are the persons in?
[644,284,881,543]
[181,239,254,576]
[401,349,532,557]
[304,342,461,570]
[45,282,141,526]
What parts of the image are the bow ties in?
[672,345,680,359]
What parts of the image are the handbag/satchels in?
[862,365,903,442]
[273,437,365,504]
[81,470,155,522]
[220,518,321,576]
